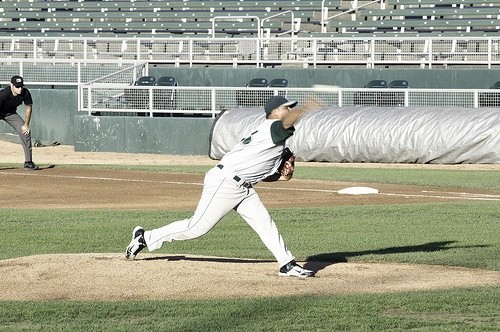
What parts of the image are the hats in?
[11,75,25,87]
[264,95,298,113]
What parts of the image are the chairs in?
[354,80,408,106]
[484,81,500,107]
[236,79,288,107]
[125,76,177,109]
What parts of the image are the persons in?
[0,75,39,170]
[125,96,328,277]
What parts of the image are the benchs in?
[0,0,500,62]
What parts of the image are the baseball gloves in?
[277,146,296,181]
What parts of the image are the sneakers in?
[125,226,146,261]
[23,162,39,170]
[278,262,315,278]
